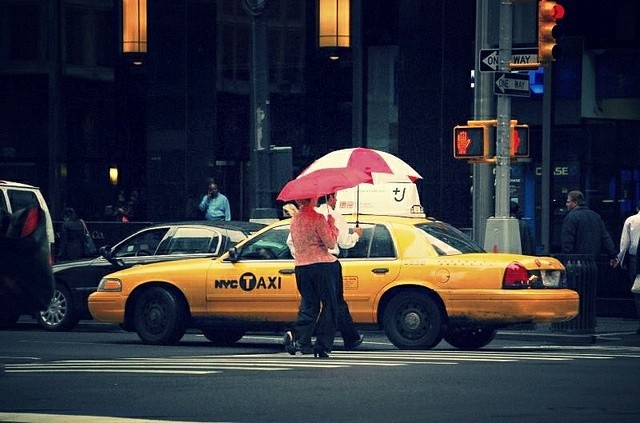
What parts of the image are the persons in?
[549,190,619,330]
[611,195,640,335]
[198,183,231,221]
[510,200,533,254]
[55,203,97,261]
[286,190,364,354]
[283,197,339,357]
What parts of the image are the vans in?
[1,180,54,324]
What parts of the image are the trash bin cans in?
[554,253,596,333]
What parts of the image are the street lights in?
[110,165,118,221]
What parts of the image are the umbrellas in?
[296,146,424,226]
[276,166,373,214]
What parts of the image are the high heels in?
[283,331,297,355]
[313,344,330,358]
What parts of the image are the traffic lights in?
[509,125,529,157]
[538,1,564,59]
[453,125,485,159]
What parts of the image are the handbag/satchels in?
[81,219,97,256]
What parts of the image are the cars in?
[90,215,579,348]
[38,221,268,330]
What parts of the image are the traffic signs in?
[493,72,529,99]
[480,48,537,71]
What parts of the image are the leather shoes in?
[295,341,312,353]
[346,334,364,350]
